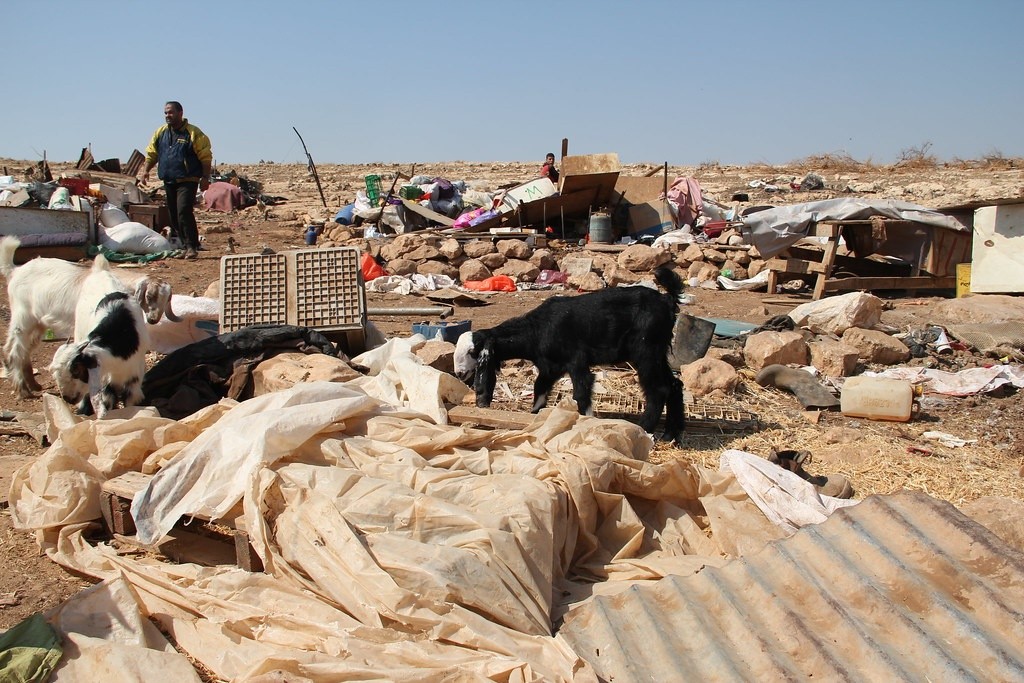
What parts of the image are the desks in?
[768,220,957,302]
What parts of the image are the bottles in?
[305,226,317,246]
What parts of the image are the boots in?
[666,313,716,375]
[755,364,841,411]
[768,450,852,499]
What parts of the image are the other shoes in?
[185,249,198,259]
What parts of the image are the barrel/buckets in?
[840,375,923,421]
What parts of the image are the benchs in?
[0,206,91,265]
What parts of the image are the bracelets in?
[203,176,210,178]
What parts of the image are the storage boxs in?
[365,174,385,207]
[627,198,677,240]
[129,204,170,233]
[399,185,423,199]
[88,183,122,209]
[58,177,90,196]
[489,226,547,247]
[219,246,367,354]
[412,320,471,343]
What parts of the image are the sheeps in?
[453,268,686,442]
[0,234,184,401]
[47,253,149,420]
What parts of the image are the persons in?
[541,153,559,184]
[141,102,212,259]
